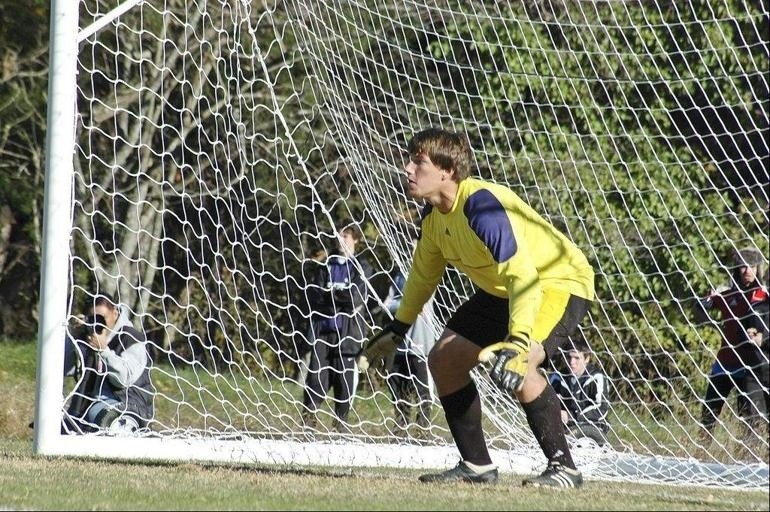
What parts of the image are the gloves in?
[475,339,530,393]
[355,326,406,376]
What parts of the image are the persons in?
[28,292,156,436]
[360,129,596,492]
[304,220,370,437]
[697,248,770,456]
[382,237,435,444]
[546,336,610,447]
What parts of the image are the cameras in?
[86,314,106,333]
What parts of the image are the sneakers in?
[520,463,584,494]
[418,459,501,489]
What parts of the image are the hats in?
[732,245,764,283]
